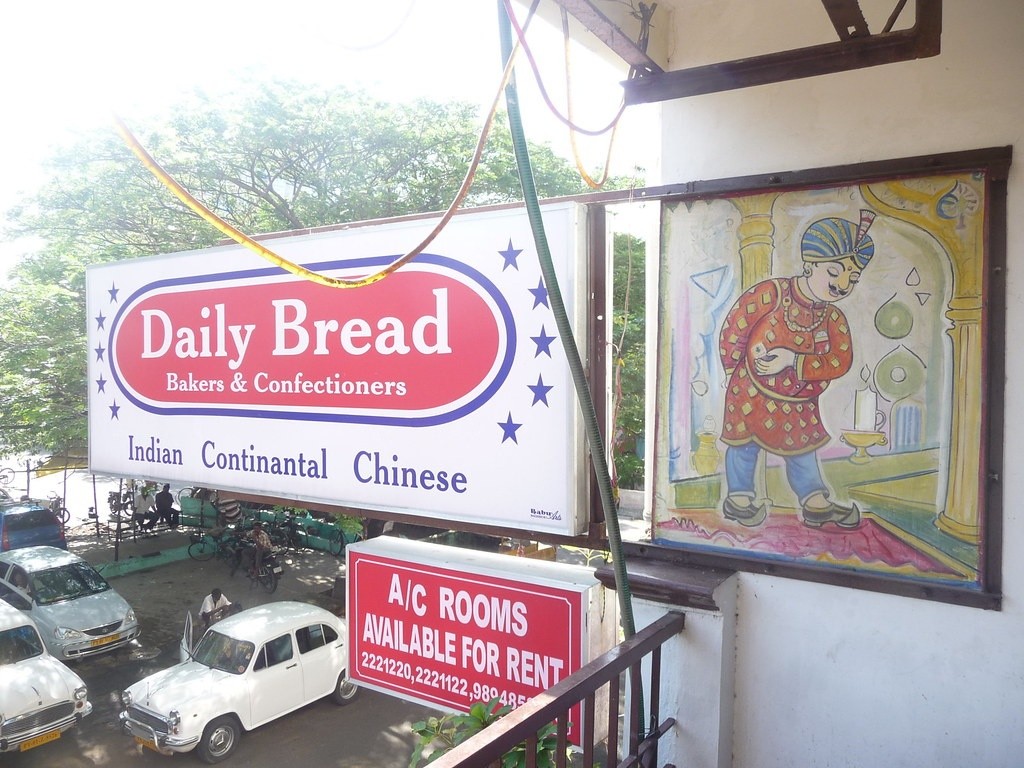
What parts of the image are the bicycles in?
[175,487,364,561]
[47,491,70,524]
[107,484,137,516]
[0,465,15,485]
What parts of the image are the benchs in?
[107,510,184,543]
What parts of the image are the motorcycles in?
[229,537,285,594]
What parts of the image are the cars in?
[118,601,360,763]
[0,504,142,752]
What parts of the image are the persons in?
[199,589,233,632]
[156,485,179,529]
[241,644,273,670]
[243,523,273,579]
[133,487,159,530]
